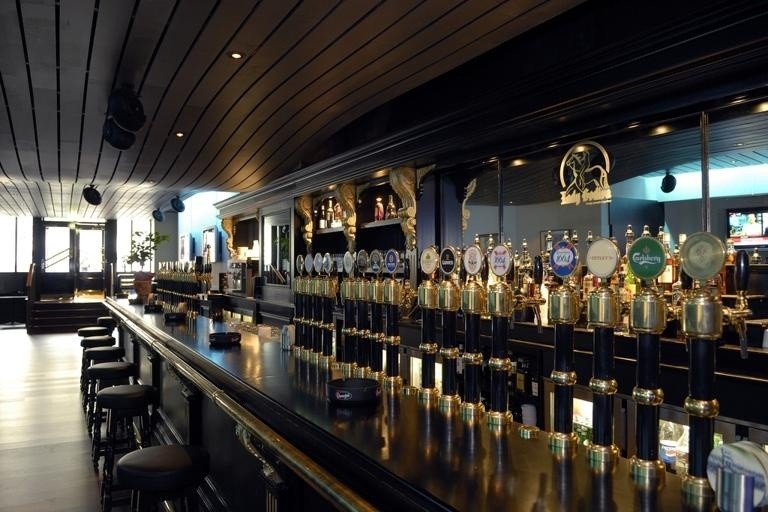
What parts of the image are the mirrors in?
[263,206,292,288]
[231,215,259,262]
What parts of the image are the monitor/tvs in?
[726,205,768,239]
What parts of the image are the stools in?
[95,385,157,510]
[76,326,124,428]
[117,441,211,511]
[86,361,136,442]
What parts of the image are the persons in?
[743,214,761,236]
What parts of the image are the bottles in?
[572,423,593,446]
[725,240,736,263]
[751,247,761,264]
[158,261,208,296]
[476,225,686,336]
[314,199,334,229]
[375,198,384,219]
[282,324,293,350]
[387,195,397,219]
[331,252,408,275]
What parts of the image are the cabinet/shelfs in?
[296,168,415,274]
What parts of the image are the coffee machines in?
[225,259,246,292]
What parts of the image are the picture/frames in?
[178,233,192,260]
[203,225,220,263]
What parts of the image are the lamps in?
[152,208,163,222]
[80,185,101,204]
[102,86,148,150]
[170,195,185,211]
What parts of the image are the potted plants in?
[127,230,170,295]
